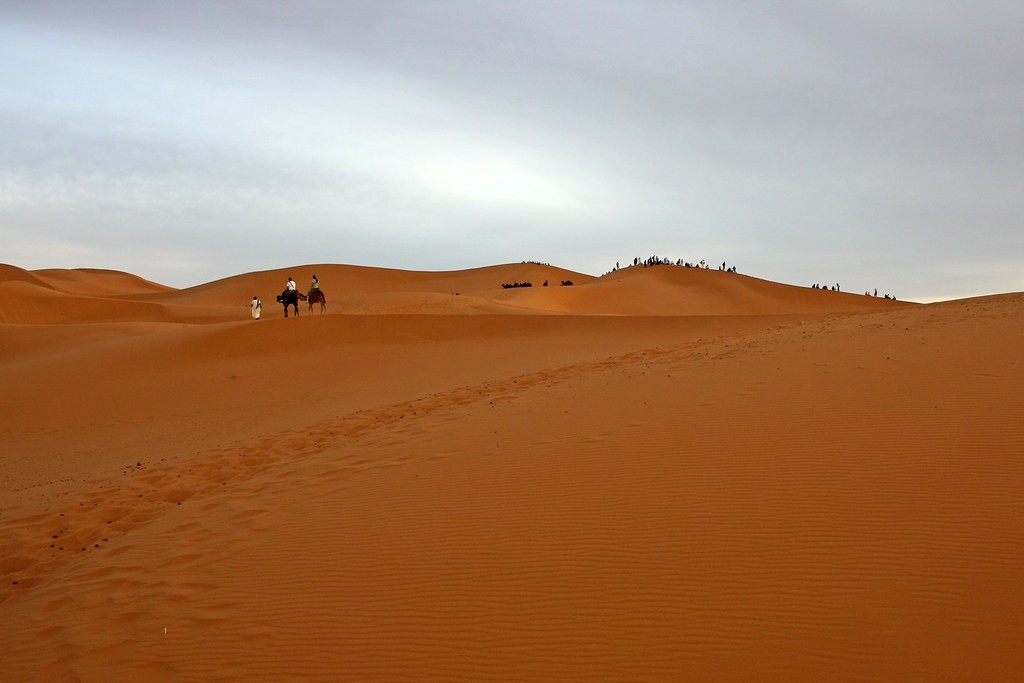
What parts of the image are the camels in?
[277,290,326,317]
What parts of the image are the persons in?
[613,255,736,272]
[305,275,319,303]
[283,277,296,304]
[251,296,263,320]
[812,283,896,301]
